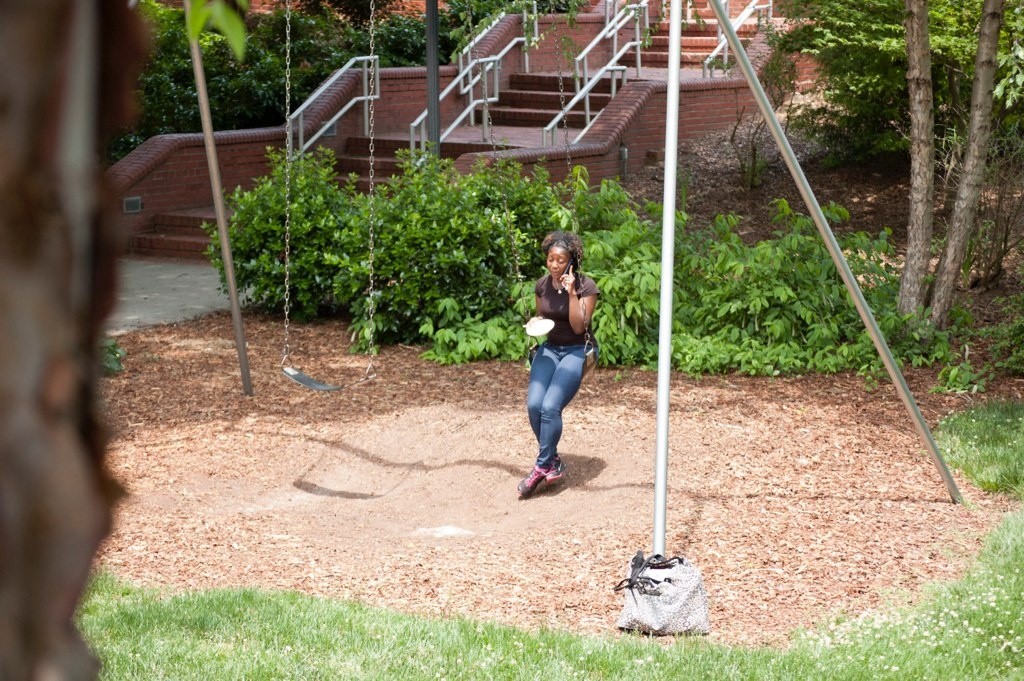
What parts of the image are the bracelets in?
[537,316,544,320]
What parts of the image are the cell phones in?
[563,259,577,274]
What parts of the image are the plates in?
[526,318,555,336]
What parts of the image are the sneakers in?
[546,456,566,484]
[518,465,544,496]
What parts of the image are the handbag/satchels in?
[615,550,709,636]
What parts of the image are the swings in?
[464,1,598,380]
[277,1,380,393]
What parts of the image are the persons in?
[517,231,600,498]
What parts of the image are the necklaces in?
[554,278,563,294]
